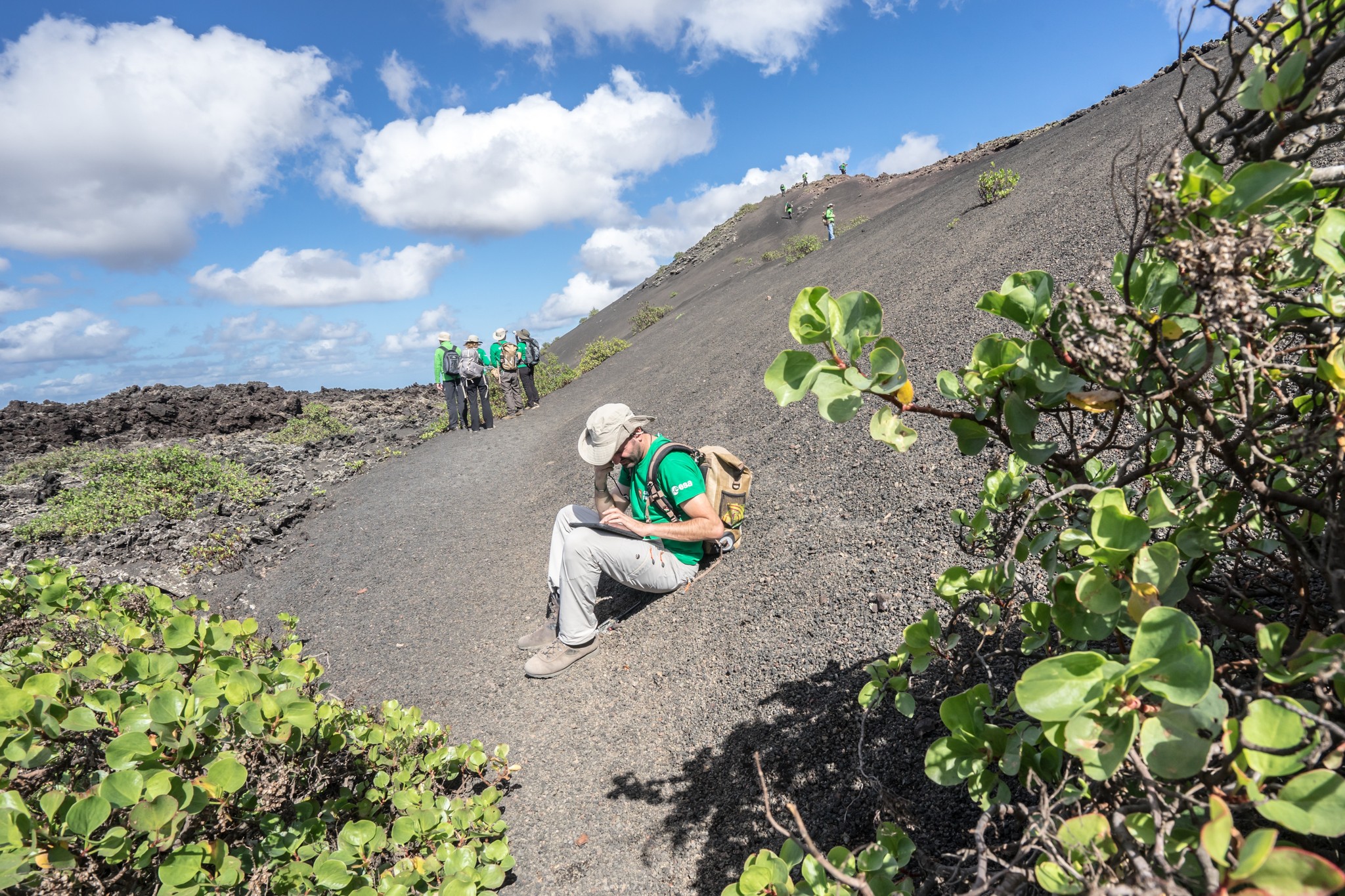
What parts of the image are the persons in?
[780,184,786,197]
[512,328,540,410]
[802,172,808,188]
[460,335,496,432]
[517,402,725,679]
[434,331,468,436]
[490,328,524,420]
[785,201,793,220]
[826,203,835,240]
[840,163,845,174]
[844,164,848,174]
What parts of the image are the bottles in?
[512,331,520,338]
[500,352,504,362]
[516,353,519,363]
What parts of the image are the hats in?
[806,172,808,174]
[842,163,848,165]
[437,332,450,341]
[463,335,483,343]
[577,402,658,465]
[516,328,531,339]
[827,203,835,209]
[493,328,508,340]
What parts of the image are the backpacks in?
[781,184,784,188]
[822,211,830,226]
[787,202,791,207]
[839,165,843,170]
[516,337,540,368]
[456,347,489,398]
[436,344,460,391]
[492,342,519,384]
[627,433,754,585]
[802,174,805,178]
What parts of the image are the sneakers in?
[516,595,560,650]
[524,633,602,679]
[445,402,542,433]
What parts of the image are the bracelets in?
[594,480,607,493]
[515,338,518,339]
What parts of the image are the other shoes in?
[782,195,785,197]
[789,216,792,220]
[803,186,808,188]
[831,238,835,241]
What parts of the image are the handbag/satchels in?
[490,367,499,380]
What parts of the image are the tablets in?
[568,523,644,541]
